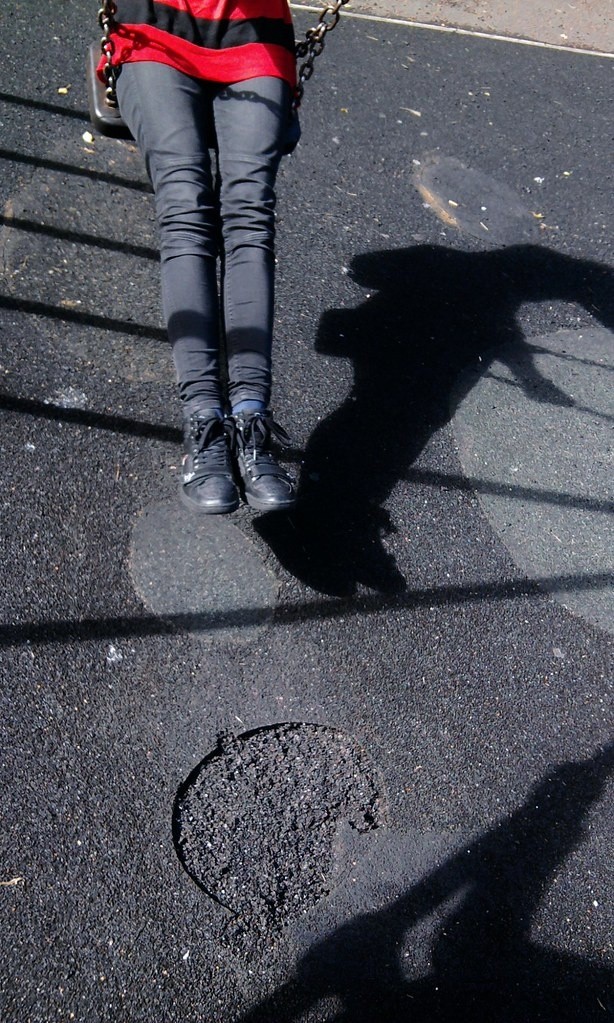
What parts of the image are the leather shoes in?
[227,408,296,510]
[179,409,240,515]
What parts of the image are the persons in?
[96,0,297,516]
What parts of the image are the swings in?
[86,0,350,157]
